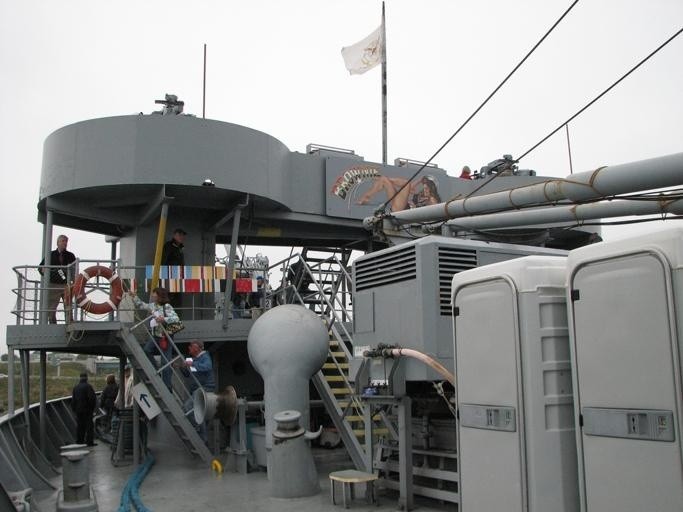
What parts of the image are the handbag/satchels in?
[160,316,184,336]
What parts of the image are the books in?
[185,357,196,372]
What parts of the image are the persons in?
[98,373,118,434]
[124,286,182,396]
[283,254,314,304]
[158,229,188,319]
[70,372,99,447]
[171,337,215,446]
[459,166,472,180]
[37,235,79,324]
[124,364,130,385]
[420,176,442,204]
[230,275,265,319]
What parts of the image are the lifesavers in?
[74,266,123,314]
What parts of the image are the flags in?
[339,25,383,76]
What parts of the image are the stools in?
[329,469,378,509]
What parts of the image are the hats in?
[174,229,187,235]
